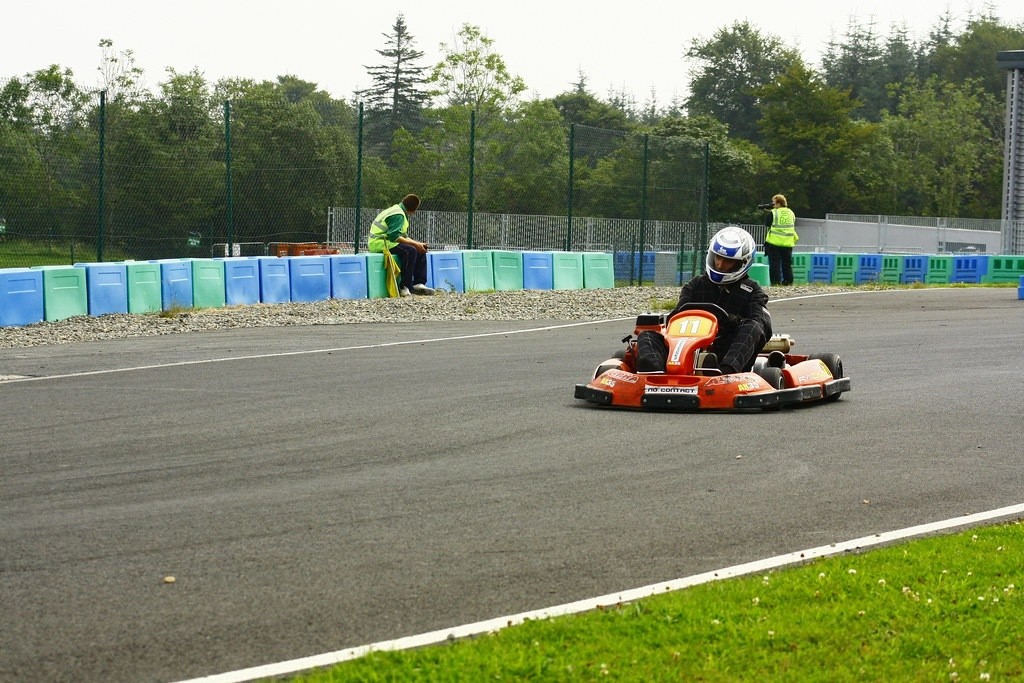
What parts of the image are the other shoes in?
[412,283,436,295]
[399,286,411,297]
[636,356,659,373]
[702,353,720,377]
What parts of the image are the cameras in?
[758,203,774,209]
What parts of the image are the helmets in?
[706,225,756,285]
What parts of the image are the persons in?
[764,194,800,286]
[368,194,437,298]
[633,225,772,377]
